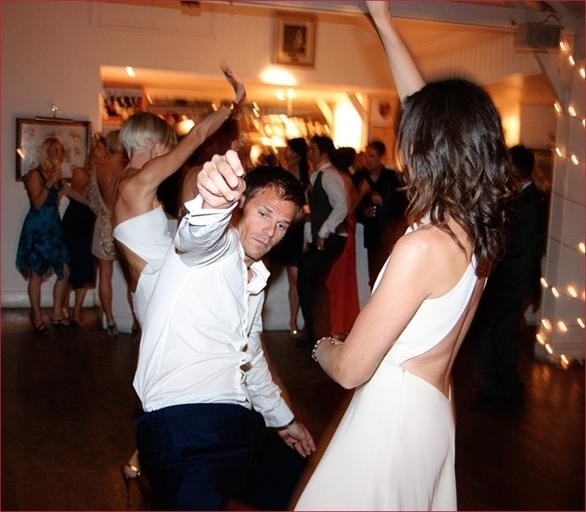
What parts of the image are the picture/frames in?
[13,115,93,185]
[272,10,318,69]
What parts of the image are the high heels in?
[122,462,150,507]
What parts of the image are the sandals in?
[106,323,119,338]
[35,307,85,334]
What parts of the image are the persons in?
[112,60,246,503]
[14,132,549,414]
[128,149,317,511]
[295,0,517,511]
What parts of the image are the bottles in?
[102,94,144,119]
[367,201,381,219]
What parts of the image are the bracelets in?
[223,101,239,114]
